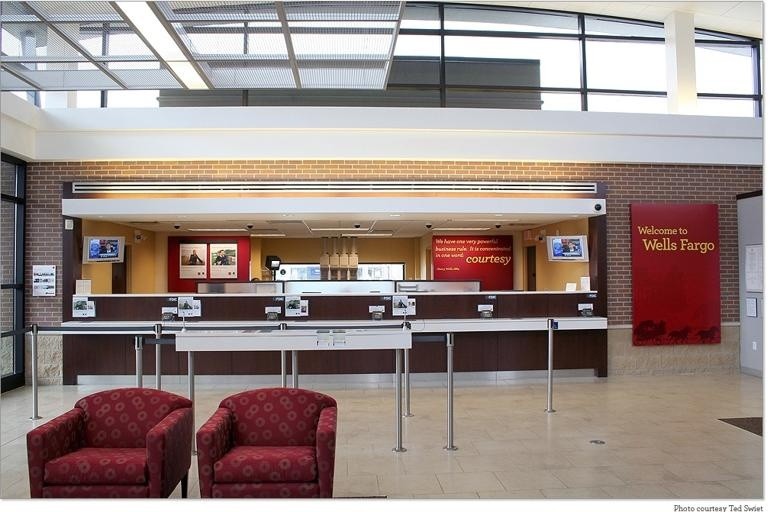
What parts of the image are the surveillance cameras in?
[425,224,432,228]
[174,225,181,229]
[354,224,361,228]
[247,224,254,229]
[495,224,502,228]
[593,203,602,212]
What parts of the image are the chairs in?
[26,387,194,498]
[196,387,338,498]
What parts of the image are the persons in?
[103,243,113,253]
[183,300,192,309]
[215,250,229,265]
[398,299,406,308]
[561,239,575,252]
[189,250,203,264]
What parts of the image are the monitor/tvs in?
[82,235,125,264]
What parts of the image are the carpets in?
[717,416,762,440]
